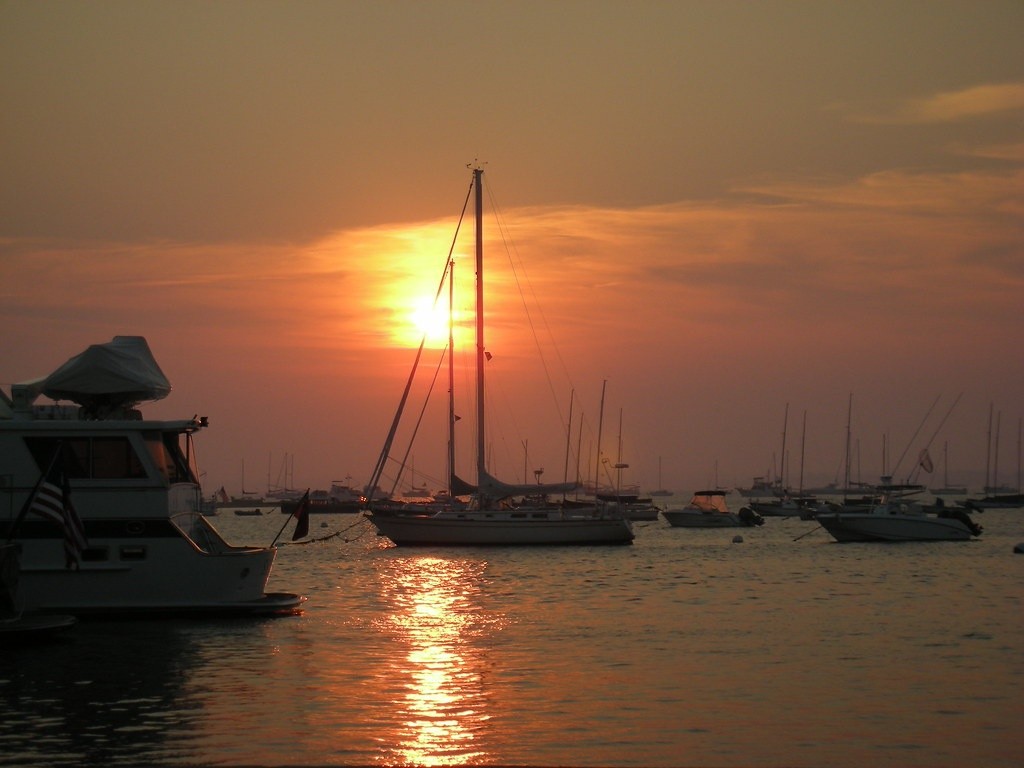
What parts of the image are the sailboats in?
[232,156,1020,548]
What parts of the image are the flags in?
[218,487,230,504]
[455,415,462,422]
[28,441,89,568]
[485,352,493,361]
[292,488,310,541]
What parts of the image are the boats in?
[0,334,310,632]
